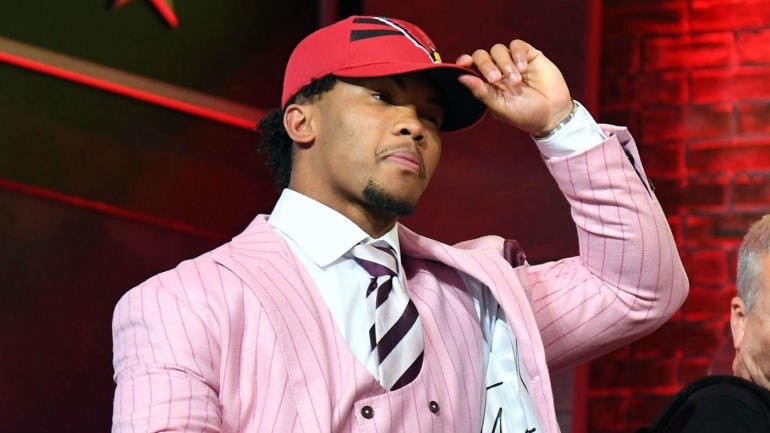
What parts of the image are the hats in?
[281,17,492,137]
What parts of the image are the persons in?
[653,214,770,433]
[113,16,689,433]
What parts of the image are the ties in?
[345,242,425,391]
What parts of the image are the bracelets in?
[534,98,579,141]
